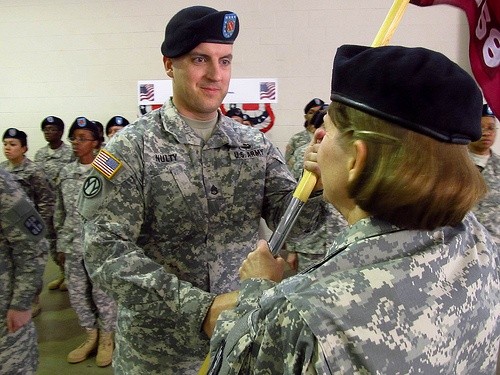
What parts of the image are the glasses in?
[69,137,96,142]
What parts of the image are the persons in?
[285,97,354,275]
[0,116,131,319]
[467,103,500,249]
[206,45,500,375]
[225,107,253,127]
[0,169,51,375]
[55,118,115,367]
[73,5,330,375]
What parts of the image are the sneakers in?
[48,276,68,290]
[30,295,41,319]
[96,332,114,367]
[68,329,98,363]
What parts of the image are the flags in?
[408,0,500,120]
[91,148,123,179]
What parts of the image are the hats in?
[69,117,98,137]
[92,121,103,131]
[305,98,324,114]
[331,45,483,145]
[310,110,327,129]
[42,116,64,129]
[228,108,243,118]
[243,114,250,121]
[483,104,493,116]
[161,6,240,59]
[3,128,27,142]
[106,116,129,135]
